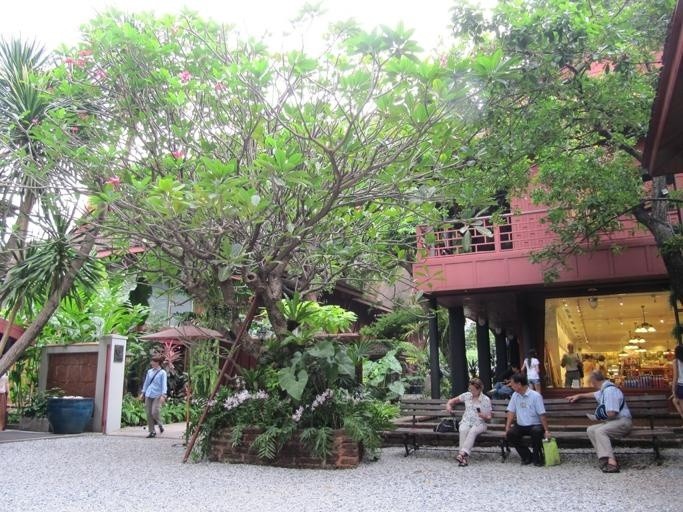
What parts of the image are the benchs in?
[383,392,676,466]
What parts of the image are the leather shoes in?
[520,453,542,467]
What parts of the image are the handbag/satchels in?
[433,415,458,432]
[541,435,562,466]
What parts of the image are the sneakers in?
[598,458,620,472]
[146,428,164,439]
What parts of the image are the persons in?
[502,373,553,467]
[671,345,682,418]
[443,379,492,465]
[560,343,584,387]
[489,362,519,400]
[596,353,608,378]
[520,348,542,394]
[138,356,167,438]
[582,355,593,386]
[565,369,632,472]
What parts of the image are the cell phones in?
[476,407,480,417]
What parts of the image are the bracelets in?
[544,430,550,433]
[672,391,674,393]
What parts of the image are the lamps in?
[617,305,656,357]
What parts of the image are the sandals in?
[456,452,468,467]
